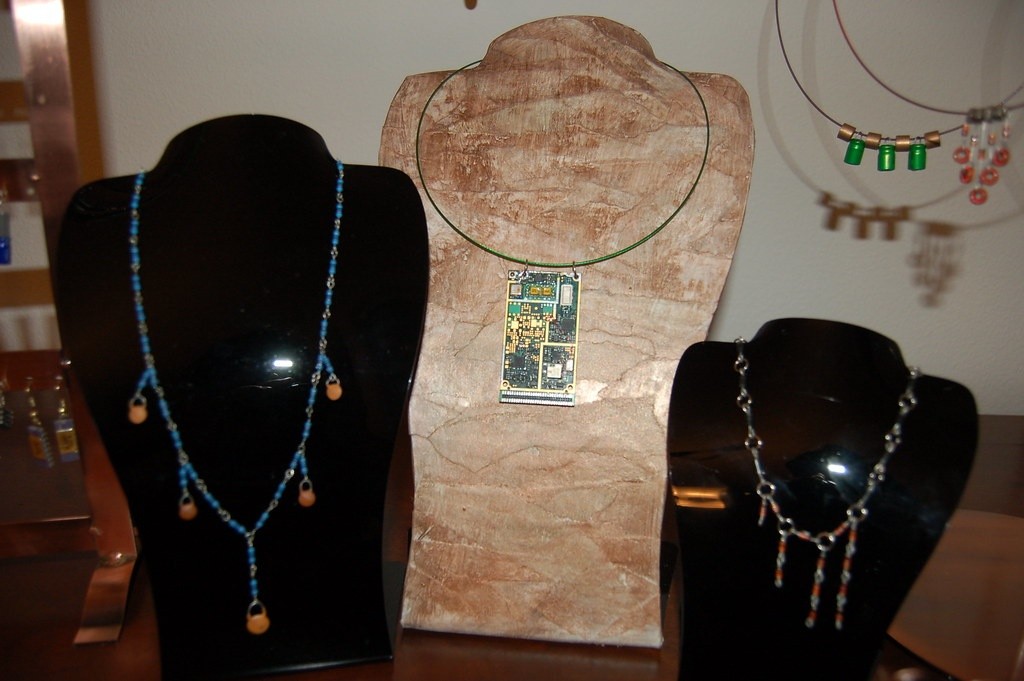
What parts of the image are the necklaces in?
[417,50,709,406]
[732,338,921,630]
[127,155,345,635]
[774,0,1024,205]
